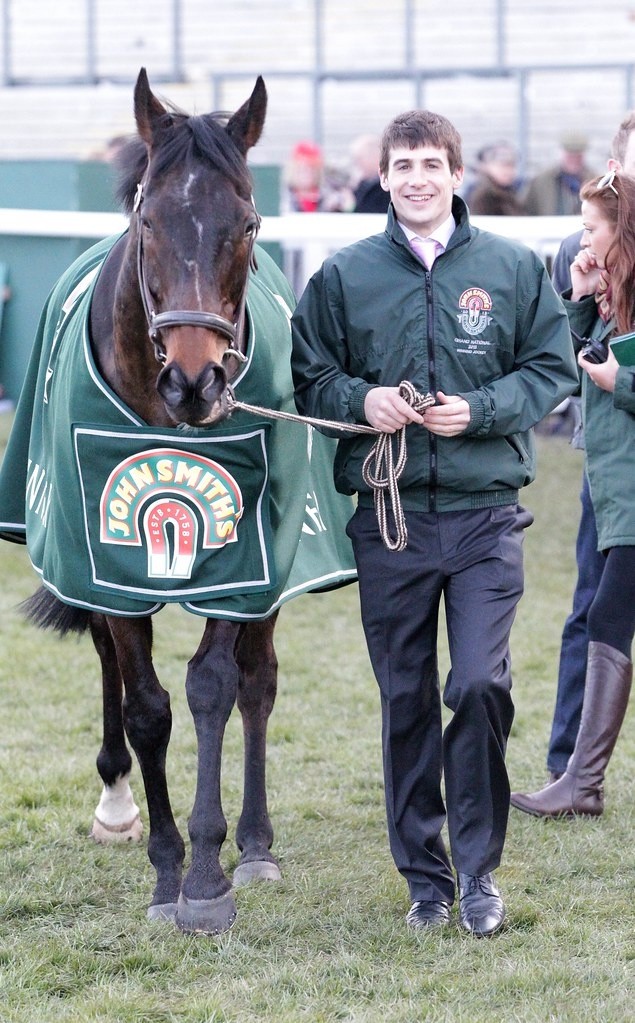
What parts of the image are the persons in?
[286,121,635,816]
[291,111,578,938]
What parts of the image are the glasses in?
[596,171,619,195]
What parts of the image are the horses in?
[12,68,295,936]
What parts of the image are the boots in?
[510,641,633,820]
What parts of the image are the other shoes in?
[550,771,563,784]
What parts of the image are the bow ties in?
[558,172,579,194]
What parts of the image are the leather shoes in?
[457,871,506,938]
[406,901,451,932]
